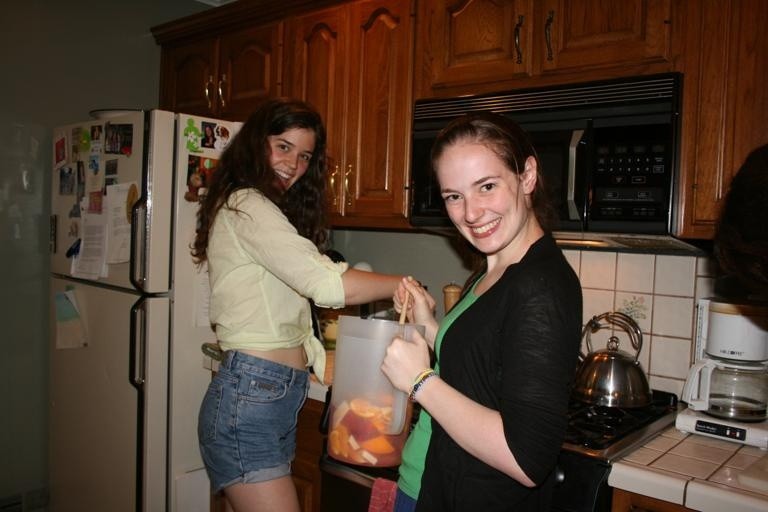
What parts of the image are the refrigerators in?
[47,110,242,511]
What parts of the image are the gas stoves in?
[563,390,679,449]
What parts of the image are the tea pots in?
[572,311,652,407]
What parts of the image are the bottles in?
[444,281,462,315]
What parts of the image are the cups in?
[327,315,425,468]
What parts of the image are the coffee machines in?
[676,298,767,452]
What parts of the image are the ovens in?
[321,389,612,511]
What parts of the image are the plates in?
[89,108,141,120]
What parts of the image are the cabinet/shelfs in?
[677,0,768,240]
[158,20,284,123]
[284,0,416,218]
[431,0,671,89]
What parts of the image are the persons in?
[202,124,216,148]
[194,96,440,512]
[380,107,584,511]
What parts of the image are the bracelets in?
[407,366,437,403]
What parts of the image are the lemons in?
[350,396,377,418]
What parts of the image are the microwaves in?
[409,73,707,256]
